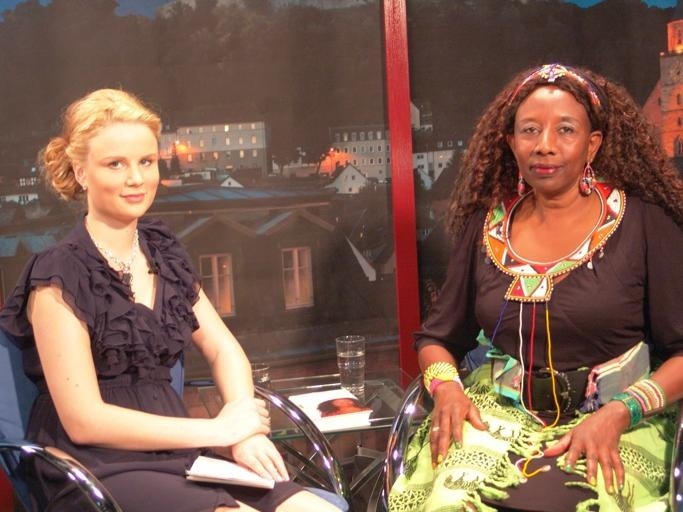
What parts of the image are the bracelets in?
[608,379,667,432]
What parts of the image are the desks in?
[194,366,429,511]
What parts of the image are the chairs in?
[381,368,682,511]
[1,330,352,512]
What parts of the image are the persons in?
[384,65,682,511]
[1,87,352,512]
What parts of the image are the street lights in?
[329,147,335,174]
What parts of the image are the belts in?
[523,367,597,416]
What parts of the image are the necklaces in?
[85,227,145,285]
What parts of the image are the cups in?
[250,362,270,420]
[336,333,365,394]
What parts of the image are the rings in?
[430,425,443,433]
